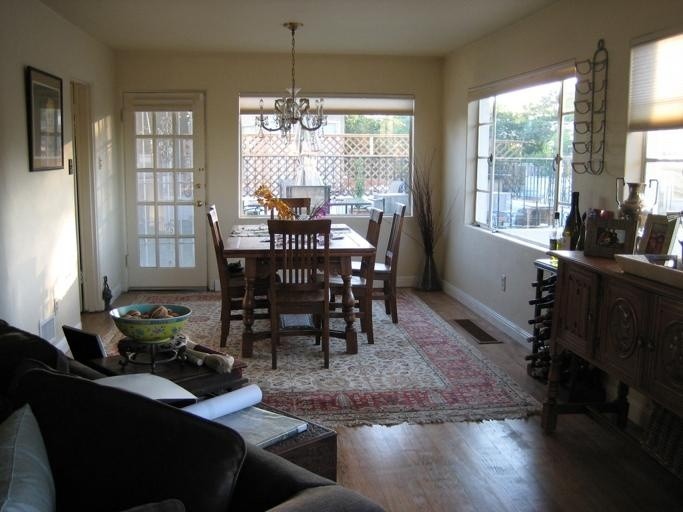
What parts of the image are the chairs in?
[206,184,407,371]
[61,325,107,360]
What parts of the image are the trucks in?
[490,191,555,232]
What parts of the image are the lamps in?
[253,22,330,133]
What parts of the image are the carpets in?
[69,287,546,424]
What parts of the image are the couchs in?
[0,318,387,512]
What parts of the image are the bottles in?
[549,213,565,260]
[564,191,582,251]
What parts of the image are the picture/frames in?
[24,66,65,172]
[636,211,680,255]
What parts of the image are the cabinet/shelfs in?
[572,38,608,178]
[539,249,683,472]
[526,258,558,384]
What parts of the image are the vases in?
[611,176,659,243]
[417,254,442,291]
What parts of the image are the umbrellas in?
[293,115,324,186]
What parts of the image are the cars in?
[241,181,404,215]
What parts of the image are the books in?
[211,406,306,449]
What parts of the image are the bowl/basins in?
[106,303,193,345]
